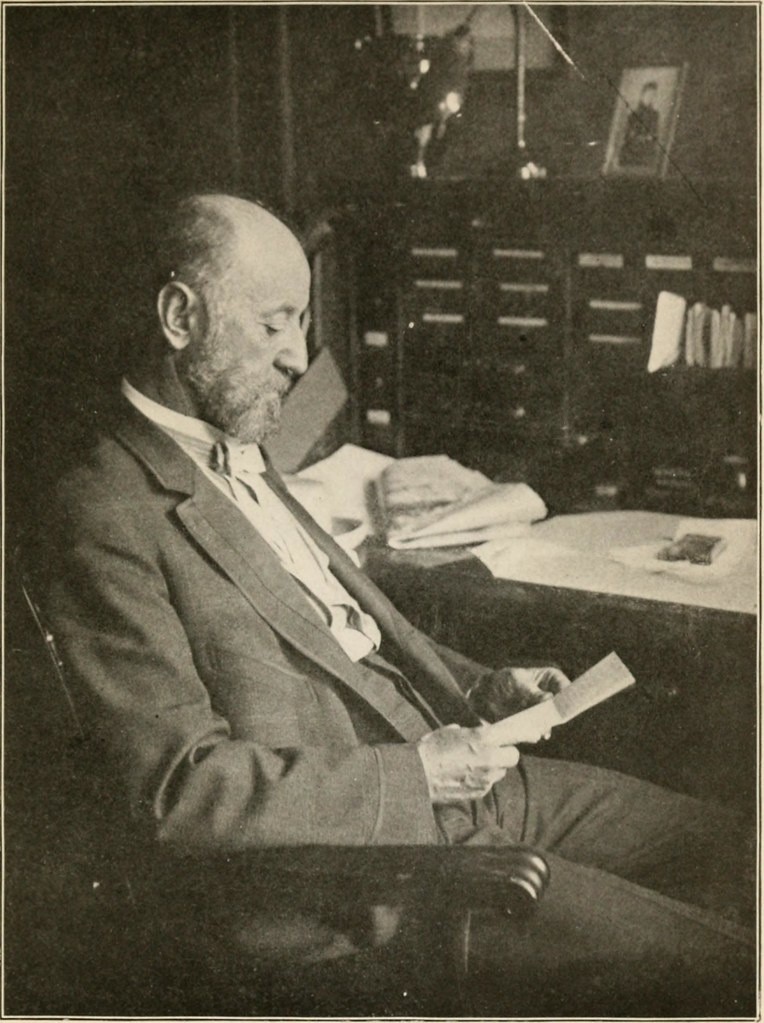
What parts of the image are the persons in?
[3,194,755,1017]
[616,79,662,173]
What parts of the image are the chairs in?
[10,513,554,1009]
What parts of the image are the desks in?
[367,540,757,812]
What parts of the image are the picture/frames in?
[600,59,688,184]
